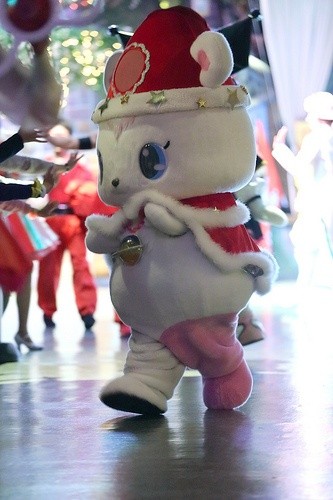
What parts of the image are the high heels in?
[14,332,43,352]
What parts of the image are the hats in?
[303,90,333,121]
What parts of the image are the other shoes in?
[43,315,55,327]
[82,315,95,329]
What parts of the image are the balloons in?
[0,0,62,78]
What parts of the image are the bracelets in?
[64,163,69,172]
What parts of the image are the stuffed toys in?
[84,7,279,415]
[232,153,288,346]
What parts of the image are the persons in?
[0,152,84,350]
[271,92,333,411]
[0,125,55,200]
[37,119,98,329]
[71,168,130,336]
